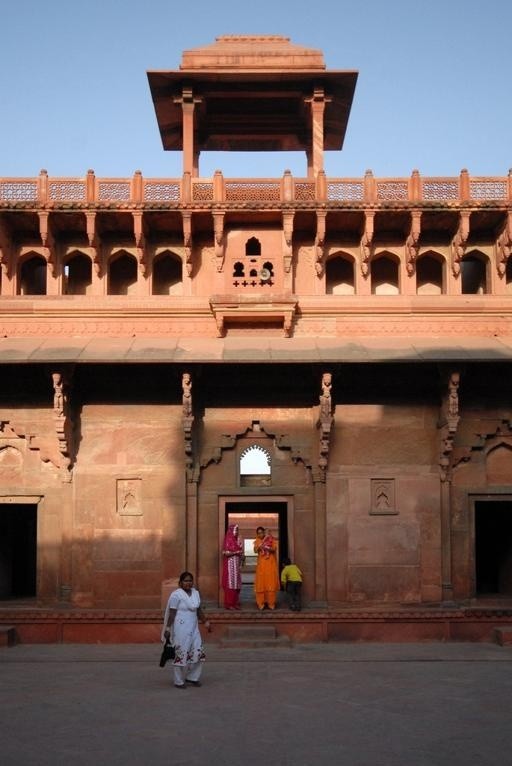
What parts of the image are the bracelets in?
[204,620,210,627]
[166,626,172,633]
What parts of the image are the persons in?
[160,571,214,691]
[259,528,274,561]
[252,526,280,612]
[222,523,244,611]
[278,557,304,612]
